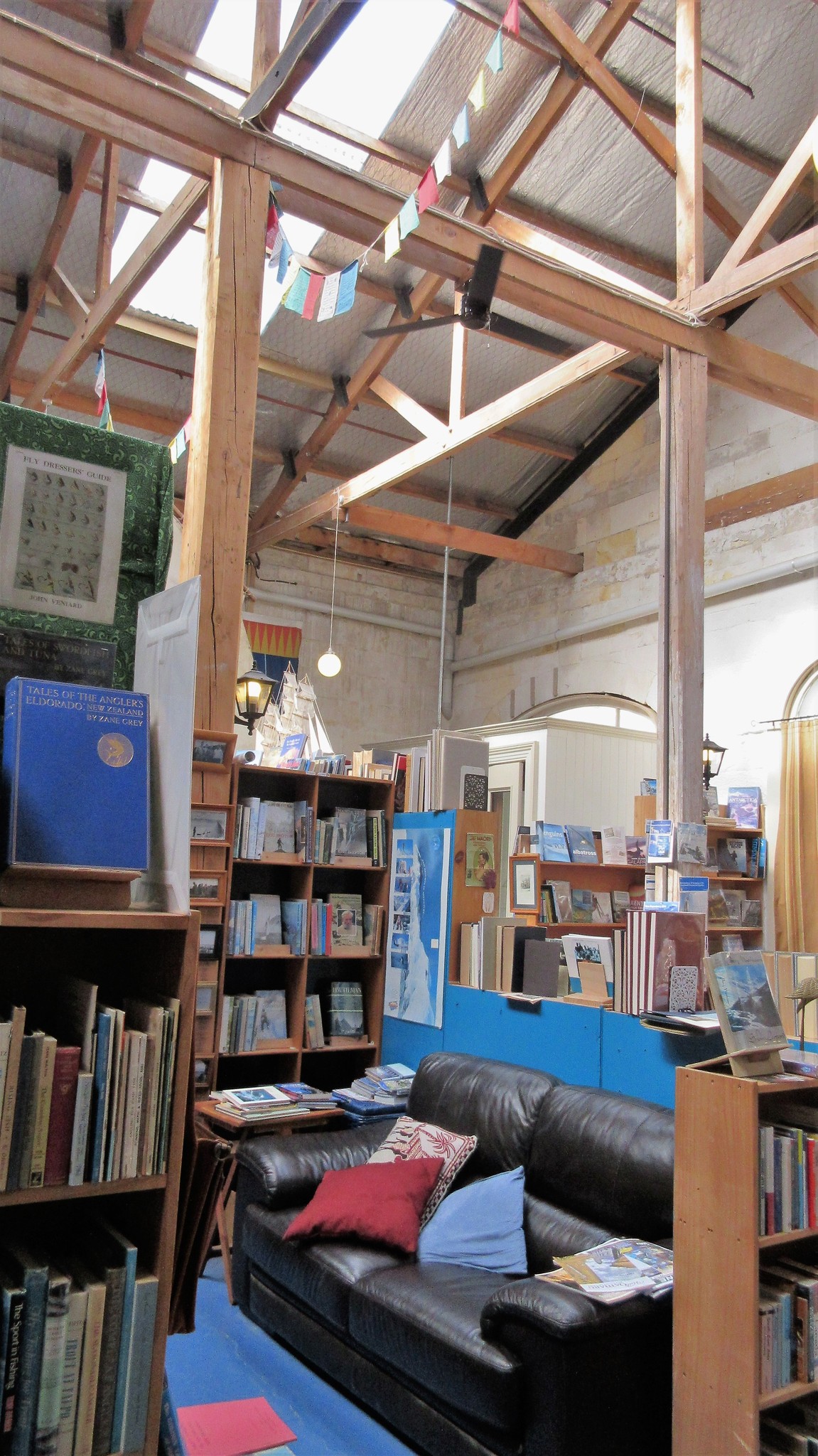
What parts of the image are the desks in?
[196,1090,342,1305]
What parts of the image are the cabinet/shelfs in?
[639,794,766,952]
[676,1047,817,1455]
[218,766,393,1084]
[0,910,198,1456]
[516,836,653,926]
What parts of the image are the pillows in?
[419,1166,527,1276]
[284,1158,445,1252]
[369,1116,478,1227]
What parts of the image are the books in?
[0,978,180,1192]
[757,1118,817,1236]
[207,1062,417,1122]
[757,1257,815,1395]
[0,1220,159,1456]
[534,1236,673,1305]
[216,729,818,1054]
[758,1401,818,1456]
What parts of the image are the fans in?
[360,246,507,342]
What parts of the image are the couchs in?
[228,1052,674,1456]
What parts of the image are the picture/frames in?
[188,870,226,907]
[189,802,235,847]
[196,981,217,1014]
[198,924,224,961]
[192,728,238,774]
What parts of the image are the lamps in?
[236,665,277,738]
[317,495,342,678]
